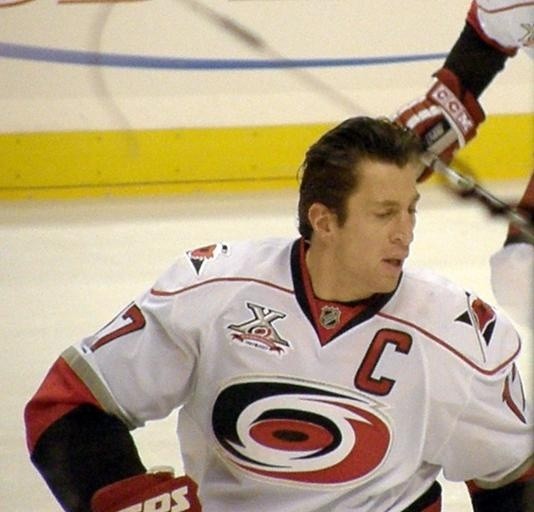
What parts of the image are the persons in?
[395,0,534,329]
[25,118,533,511]
